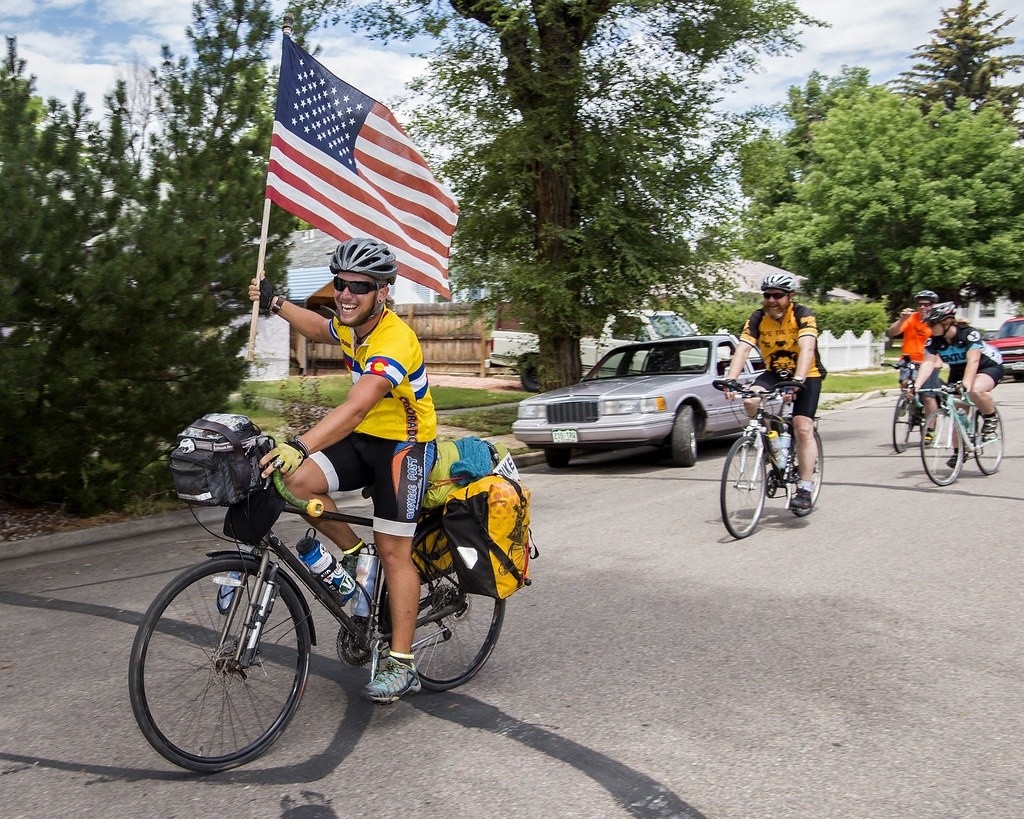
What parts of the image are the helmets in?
[329,239,398,285]
[920,302,955,323]
[913,290,939,303]
[762,275,796,291]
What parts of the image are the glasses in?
[916,303,931,307]
[762,293,786,300]
[333,276,384,294]
[925,321,937,326]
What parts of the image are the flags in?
[264,32,459,301]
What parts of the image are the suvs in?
[986,316,1024,382]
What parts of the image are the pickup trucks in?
[484,309,733,392]
[512,333,786,468]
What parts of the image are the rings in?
[273,458,283,469]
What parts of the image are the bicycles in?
[127,412,534,774]
[881,355,951,453]
[908,379,1005,487]
[713,379,824,540]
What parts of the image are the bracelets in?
[294,435,309,458]
[271,297,284,314]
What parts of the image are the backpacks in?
[444,476,532,599]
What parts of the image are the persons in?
[887,290,1005,469]
[722,274,827,510]
[249,237,438,704]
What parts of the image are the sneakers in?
[947,454,967,467]
[363,658,422,704]
[925,430,936,445]
[791,489,811,509]
[339,541,371,579]
[982,418,998,432]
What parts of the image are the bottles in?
[351,543,379,617]
[296,528,356,602]
[769,431,782,462]
[970,417,974,432]
[777,431,791,468]
[958,408,969,428]
